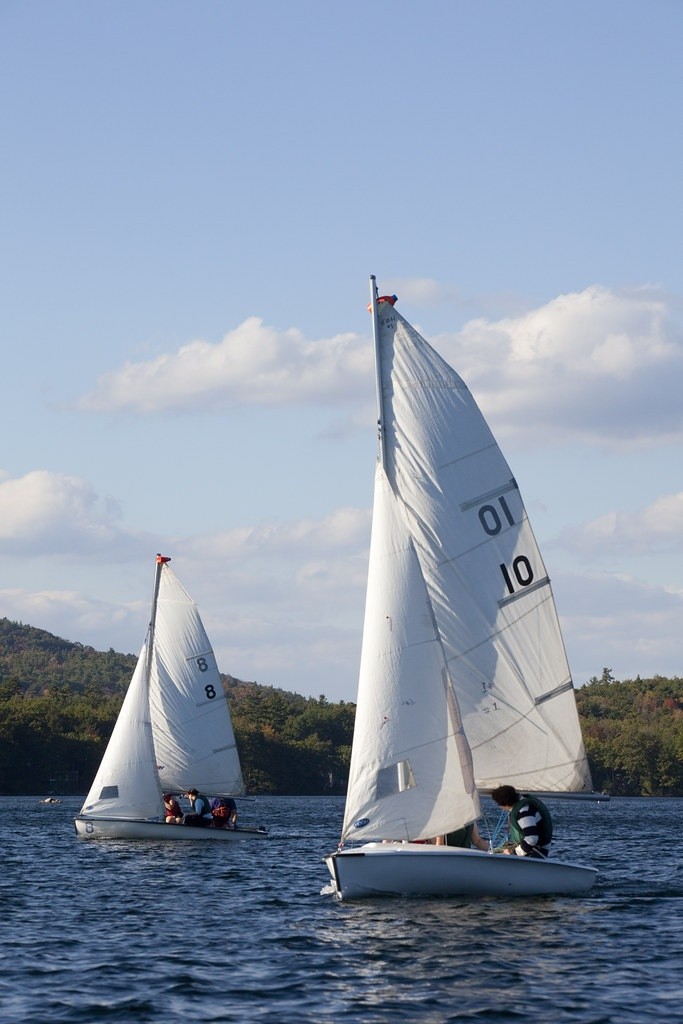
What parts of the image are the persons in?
[163,788,237,829]
[411,785,552,858]
[39,798,62,803]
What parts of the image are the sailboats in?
[317,271,602,901]
[72,553,271,841]
